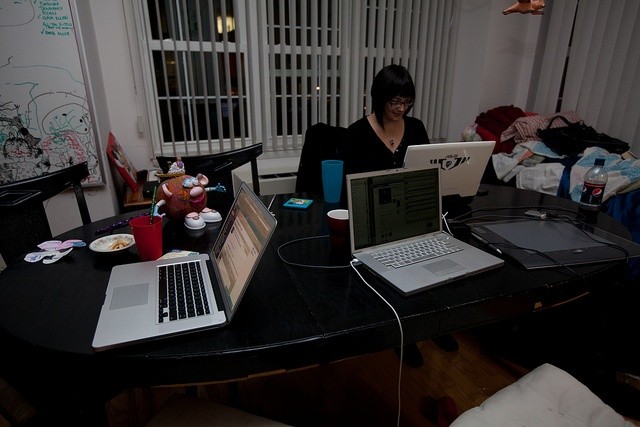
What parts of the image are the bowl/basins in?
[327,209,349,245]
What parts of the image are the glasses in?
[388,98,414,108]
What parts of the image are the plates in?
[90,234,135,252]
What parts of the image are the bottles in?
[574,159,608,224]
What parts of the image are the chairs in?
[155,141,264,207]
[449,363,633,426]
[0,160,90,266]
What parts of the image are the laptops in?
[345,162,505,295]
[92,182,279,351]
[402,139,496,203]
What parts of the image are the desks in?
[0,182,634,426]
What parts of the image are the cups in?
[129,215,162,260]
[322,159,343,203]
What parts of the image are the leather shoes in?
[432,334,460,353]
[395,342,424,369]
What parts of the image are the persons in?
[342,63,430,187]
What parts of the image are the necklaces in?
[389,139,395,146]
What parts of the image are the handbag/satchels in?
[535,116,600,156]
[575,132,631,155]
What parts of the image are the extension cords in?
[525,210,550,219]
